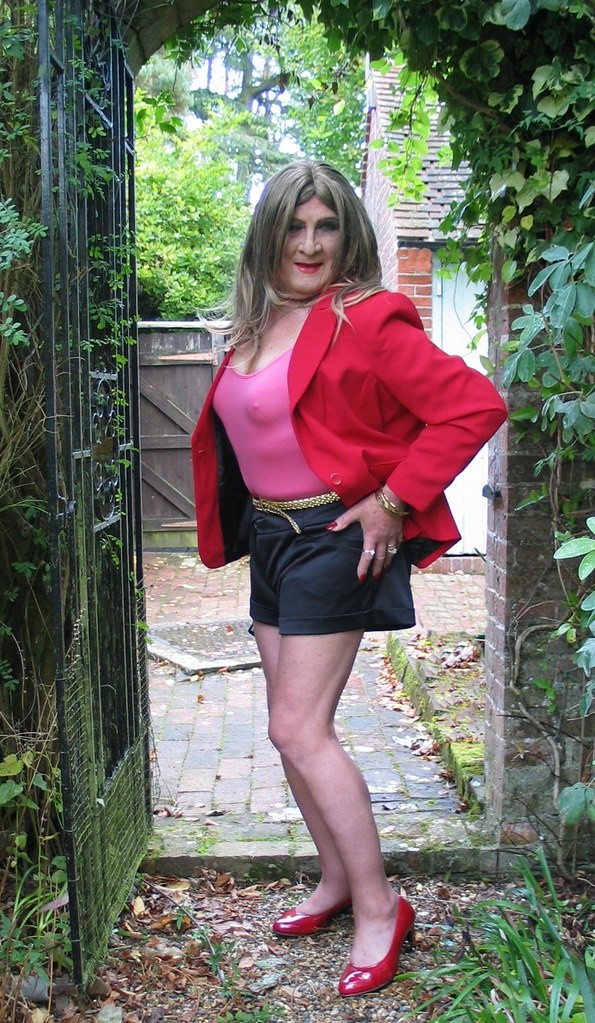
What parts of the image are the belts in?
[252,491,341,535]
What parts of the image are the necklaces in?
[258,289,324,349]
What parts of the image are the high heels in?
[338,895,416,996]
[272,895,351,936]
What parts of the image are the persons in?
[190,161,505,998]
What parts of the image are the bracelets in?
[374,487,413,520]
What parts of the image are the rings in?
[362,548,376,556]
[385,544,398,554]
